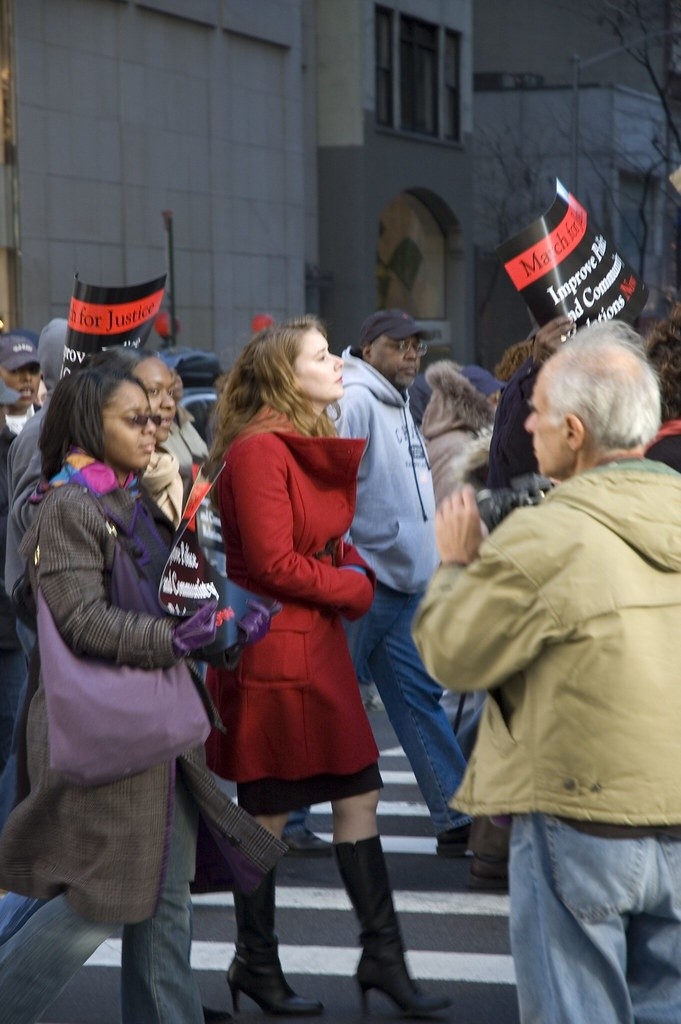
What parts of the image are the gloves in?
[235,599,282,645]
[171,598,219,657]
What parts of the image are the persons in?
[1,310,681,1024]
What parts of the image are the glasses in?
[131,415,161,427]
[399,340,427,356]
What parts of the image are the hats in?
[0,336,40,372]
[459,365,505,396]
[359,309,432,348]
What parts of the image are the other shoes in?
[471,816,511,880]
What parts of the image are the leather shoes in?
[436,823,472,857]
[281,828,332,857]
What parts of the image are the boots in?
[332,833,452,1014]
[226,866,325,1016]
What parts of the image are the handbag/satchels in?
[33,483,212,790]
